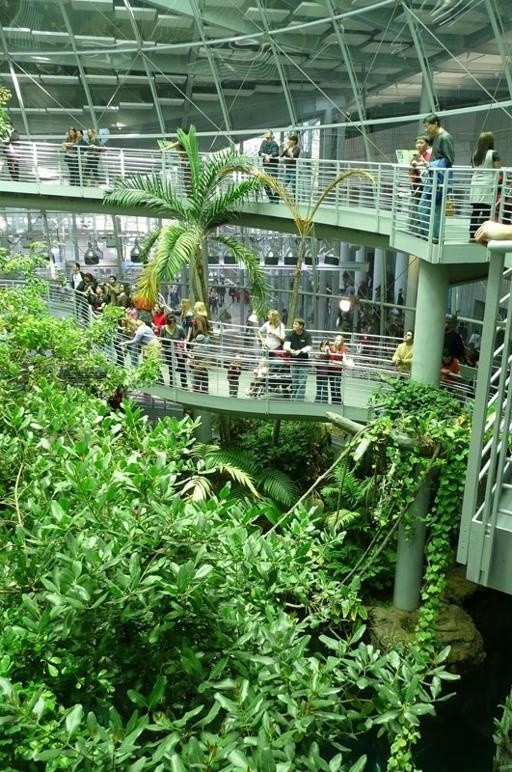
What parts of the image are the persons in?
[337,272,403,363]
[394,330,414,383]
[395,114,511,246]
[439,320,480,396]
[257,309,346,401]
[63,126,102,187]
[258,130,300,202]
[3,122,19,181]
[69,263,212,390]
[209,274,252,397]
